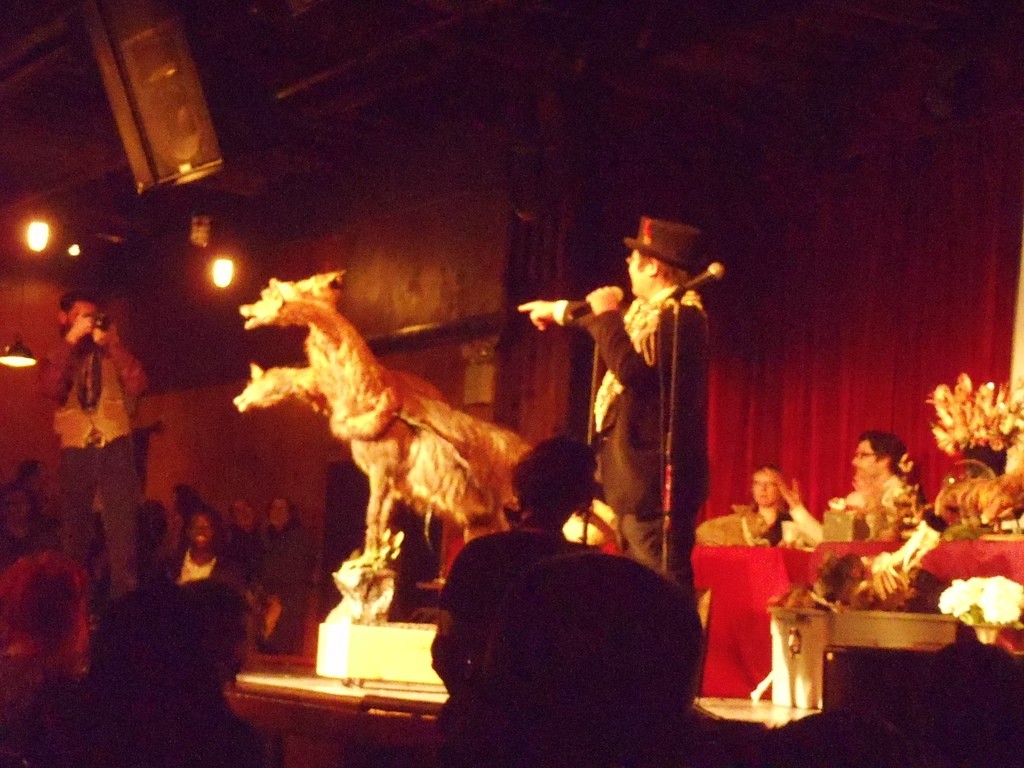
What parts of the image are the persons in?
[516,216,706,618]
[751,463,823,548]
[40,288,145,600]
[429,435,708,767]
[844,431,907,539]
[1,461,308,768]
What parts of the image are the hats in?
[623,216,707,270]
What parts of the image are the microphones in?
[668,263,725,300]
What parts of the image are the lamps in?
[0,273,38,367]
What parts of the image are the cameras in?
[88,312,110,331]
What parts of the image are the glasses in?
[856,452,884,461]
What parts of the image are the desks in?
[688,534,1024,710]
